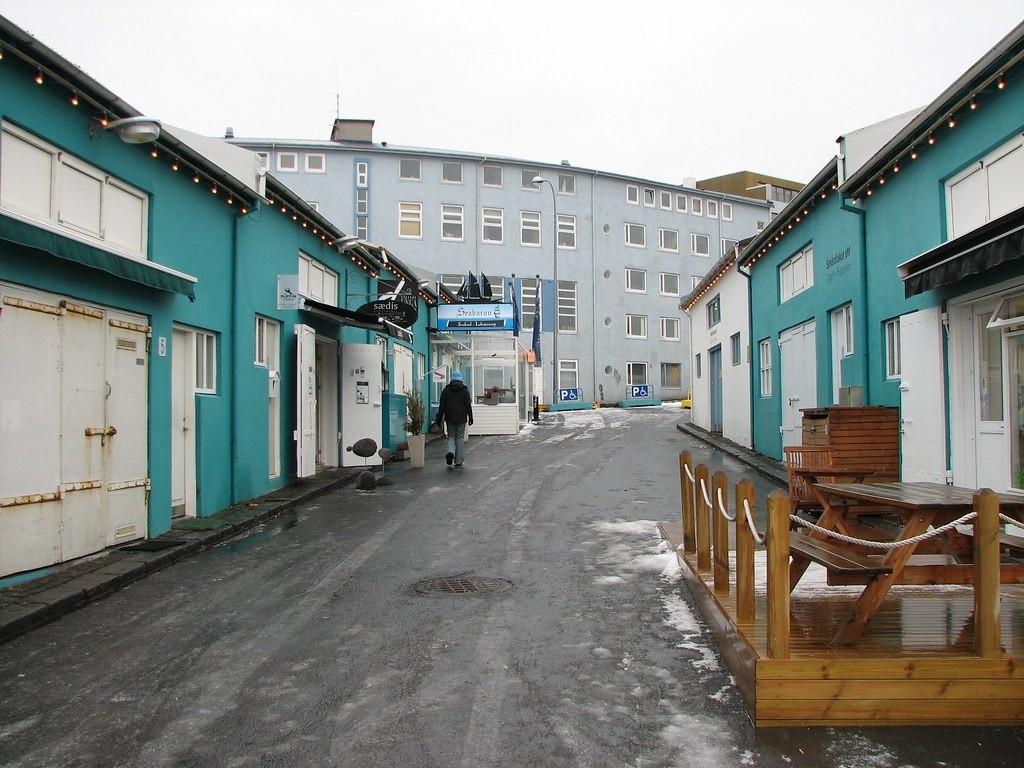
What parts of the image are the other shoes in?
[446,452,454,465]
[456,463,462,466]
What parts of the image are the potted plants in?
[484,392,491,405]
[394,441,410,461]
[490,386,499,405]
[402,390,425,468]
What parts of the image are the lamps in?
[268,198,451,304]
[32,68,248,214]
[103,116,162,144]
[852,74,1004,205]
[684,182,838,313]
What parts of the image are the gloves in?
[437,418,442,427]
[468,417,473,426]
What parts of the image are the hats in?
[452,371,462,380]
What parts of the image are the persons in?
[436,371,473,466]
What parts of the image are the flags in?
[510,286,519,336]
[532,288,541,361]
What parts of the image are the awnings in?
[298,291,413,345]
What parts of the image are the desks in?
[790,482,1023,650]
[789,466,878,521]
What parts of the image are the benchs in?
[789,528,1023,586]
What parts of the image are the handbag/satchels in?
[430,420,444,434]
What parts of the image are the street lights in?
[532,176,561,407]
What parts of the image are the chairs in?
[784,446,836,532]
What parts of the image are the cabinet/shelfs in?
[799,406,899,482]
